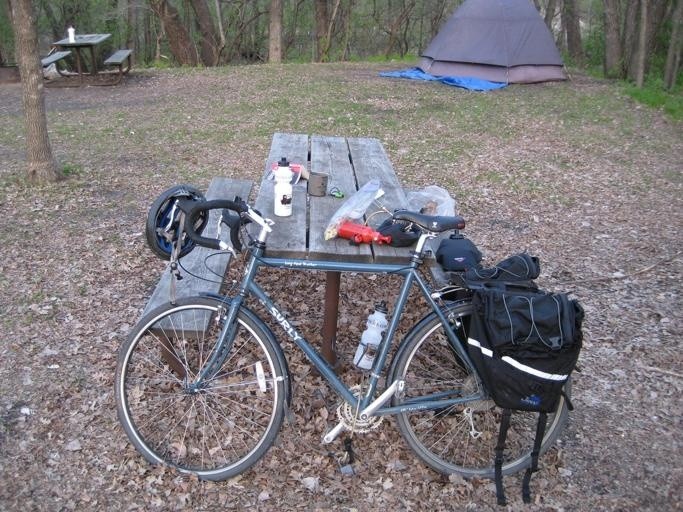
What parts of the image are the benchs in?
[41,51,72,88]
[103,49,133,86]
[134,177,255,381]
[426,231,487,376]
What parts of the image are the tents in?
[414,0,569,86]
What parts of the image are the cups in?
[307,171,333,197]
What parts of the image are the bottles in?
[273,157,294,219]
[68,26,76,44]
[352,300,390,371]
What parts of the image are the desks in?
[242,132,438,376]
[52,33,112,88]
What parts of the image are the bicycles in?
[111,192,576,487]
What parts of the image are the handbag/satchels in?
[437,253,540,368]
[467,287,578,414]
